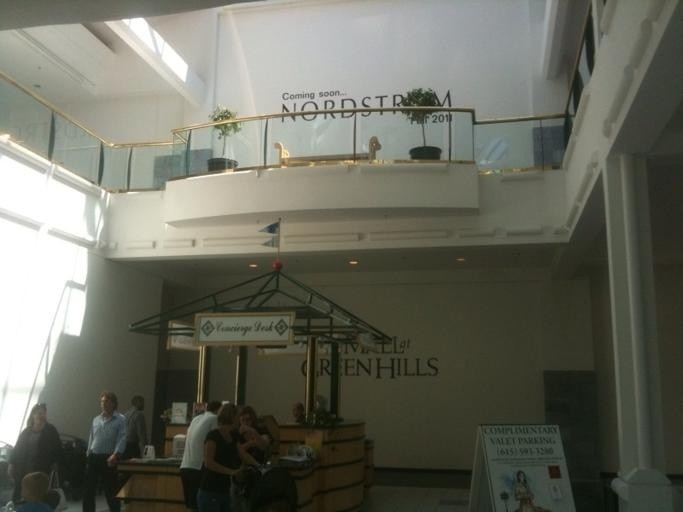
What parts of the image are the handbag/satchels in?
[54,487,67,512]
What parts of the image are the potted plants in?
[400,89,442,159]
[206,108,241,172]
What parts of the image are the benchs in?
[273,136,380,168]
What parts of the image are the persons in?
[512,470,539,512]
[15,471,53,512]
[121,395,147,504]
[197,403,259,512]
[178,401,222,512]
[291,402,306,424]
[82,391,128,512]
[7,402,62,505]
[229,406,273,512]
